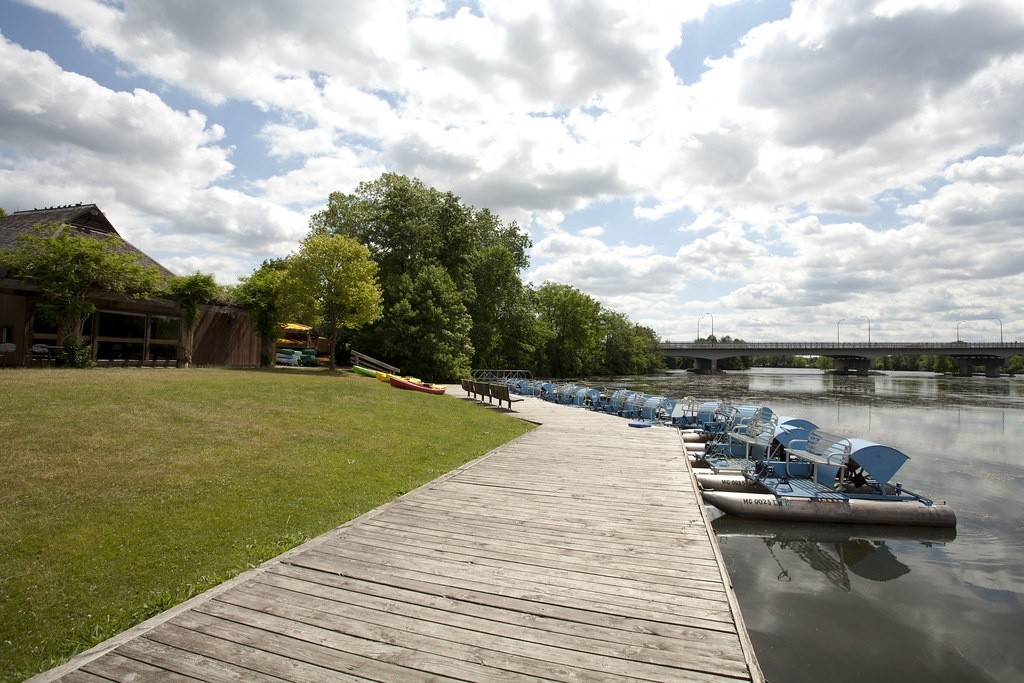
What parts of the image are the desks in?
[48,346,65,364]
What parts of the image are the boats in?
[389,376,445,395]
[353,364,377,379]
[694,431,957,529]
[375,371,436,389]
[470,368,857,475]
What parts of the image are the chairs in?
[0,343,17,368]
[25,344,50,369]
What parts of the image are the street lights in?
[697,316,705,342]
[837,319,845,343]
[861,315,871,345]
[992,318,1003,344]
[957,320,966,343]
[706,312,714,348]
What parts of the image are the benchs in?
[726,409,778,461]
[784,429,852,491]
[461,376,740,443]
